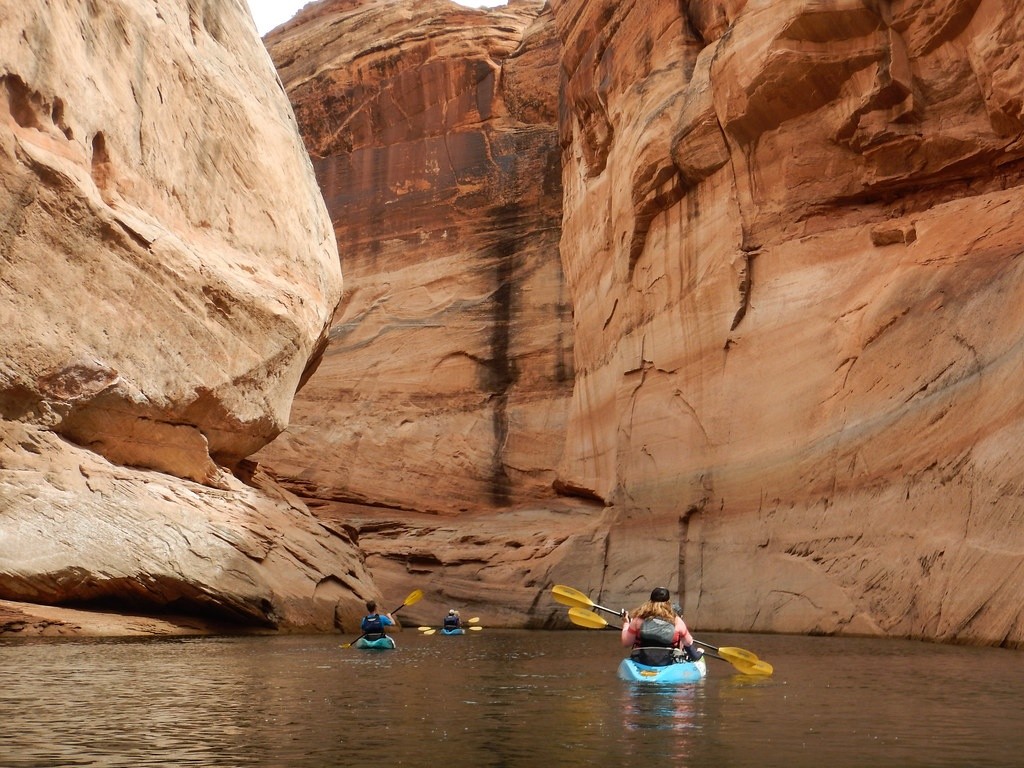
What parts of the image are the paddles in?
[417,625,483,631]
[567,606,774,674]
[551,583,759,667]
[342,589,423,648]
[425,616,480,635]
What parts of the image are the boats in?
[439,628,465,636]
[356,637,394,650]
[619,648,709,685]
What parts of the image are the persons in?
[361,601,395,641]
[444,610,462,632]
[619,586,705,667]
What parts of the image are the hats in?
[650,588,670,602]
[449,609,454,614]
